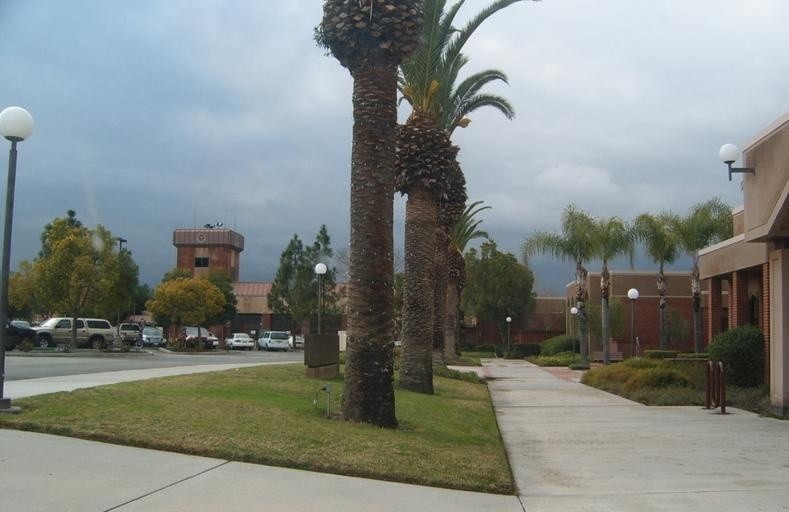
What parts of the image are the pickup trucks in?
[29,318,115,349]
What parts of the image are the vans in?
[257,331,290,352]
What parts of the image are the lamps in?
[719,144,756,181]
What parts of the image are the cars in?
[288,336,304,349]
[119,323,220,349]
[5,320,36,351]
[224,332,255,351]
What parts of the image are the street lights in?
[0,106,34,409]
[571,307,578,363]
[506,316,512,350]
[117,239,127,337]
[628,288,640,357]
[314,262,328,335]
